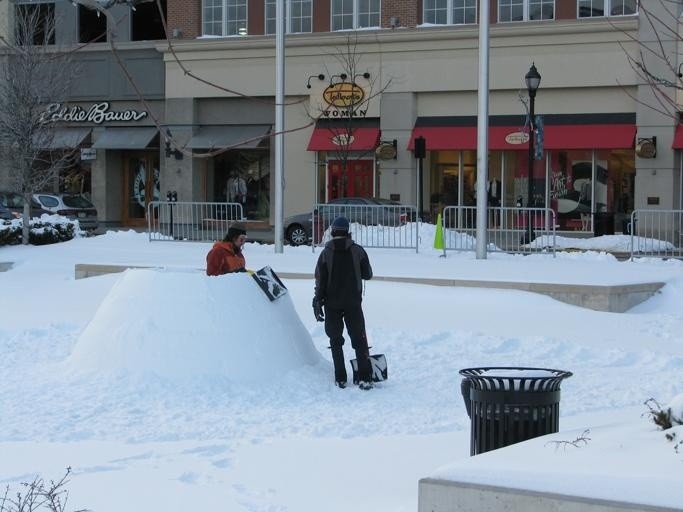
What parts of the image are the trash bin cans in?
[459,369,572,455]
[623,219,637,235]
[592,212,615,237]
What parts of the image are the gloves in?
[312,305,324,322]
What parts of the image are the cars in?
[1,193,98,229]
[284,197,416,246]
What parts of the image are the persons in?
[311,216,374,391]
[226,170,257,220]
[205,221,253,276]
[489,176,500,226]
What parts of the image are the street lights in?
[521,61,541,244]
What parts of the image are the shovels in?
[247,266,287,300]
[350,354,387,384]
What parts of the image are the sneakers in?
[334,380,347,389]
[358,382,374,391]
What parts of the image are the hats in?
[330,216,349,232]
[228,220,246,230]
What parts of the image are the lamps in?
[329,73,347,87]
[352,72,370,87]
[165,129,183,161]
[307,74,325,88]
